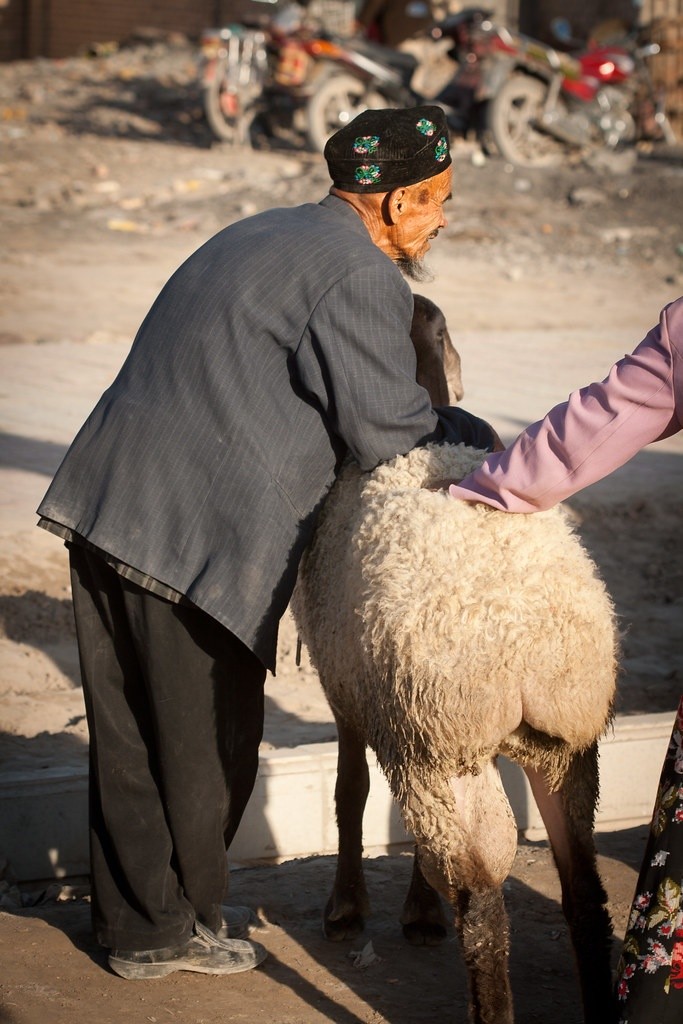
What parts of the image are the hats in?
[324,106,452,193]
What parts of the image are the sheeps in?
[287,293,620,1023]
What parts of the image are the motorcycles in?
[197,0,364,145]
[275,1,498,154]
[459,16,677,170]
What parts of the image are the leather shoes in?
[216,905,262,940]
[107,914,271,980]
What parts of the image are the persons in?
[37,105,506,982]
[633,0,683,145]
[428,296,683,1024]
